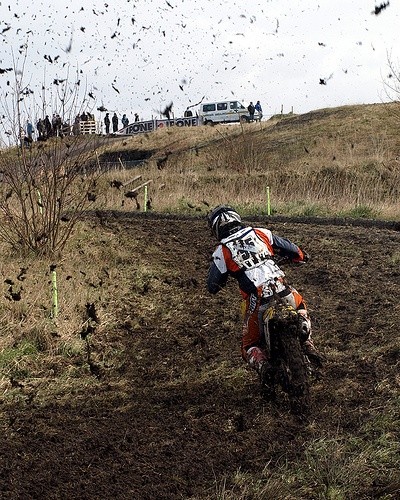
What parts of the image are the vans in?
[200,100,260,127]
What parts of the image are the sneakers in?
[307,351,325,369]
[259,361,276,389]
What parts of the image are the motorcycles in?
[257,255,308,393]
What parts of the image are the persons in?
[122,114,129,128]
[104,113,110,135]
[184,107,192,118]
[134,113,139,122]
[26,114,62,144]
[248,102,255,122]
[75,111,88,124]
[207,203,327,384]
[255,101,263,123]
[112,113,119,132]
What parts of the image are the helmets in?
[208,204,241,243]
